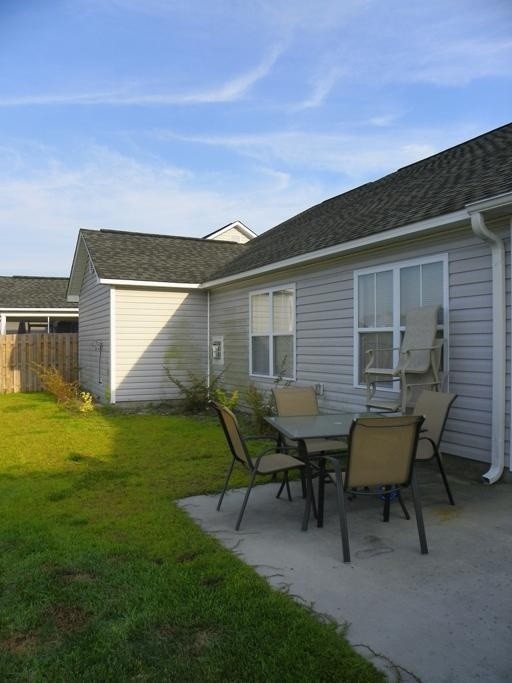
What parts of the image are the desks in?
[263,415,392,527]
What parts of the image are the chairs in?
[317,414,428,562]
[365,304,442,416]
[364,339,444,416]
[271,386,349,502]
[382,389,458,505]
[209,400,317,531]
